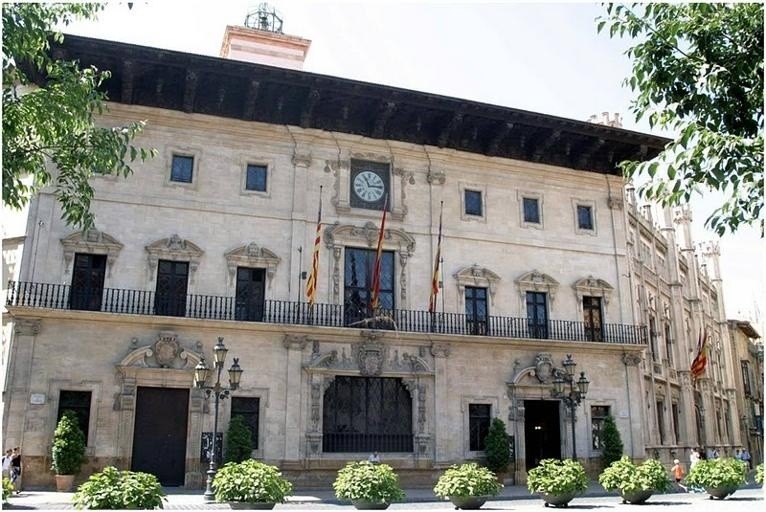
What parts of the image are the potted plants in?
[72,466,168,510]
[527,458,591,507]
[433,461,503,509]
[484,417,511,487]
[51,409,87,491]
[332,460,407,509]
[597,456,669,503]
[685,456,749,500]
[210,458,293,510]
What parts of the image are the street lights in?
[551,351,592,485]
[192,334,245,504]
[741,414,755,470]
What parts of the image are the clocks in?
[350,157,390,213]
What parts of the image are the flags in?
[691,329,707,378]
[429,201,443,313]
[305,187,322,312]
[695,331,702,357]
[369,196,387,312]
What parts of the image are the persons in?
[690,444,751,474]
[368,451,381,465]
[2,447,22,497]
[670,458,689,493]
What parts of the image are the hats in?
[674,459,679,463]
